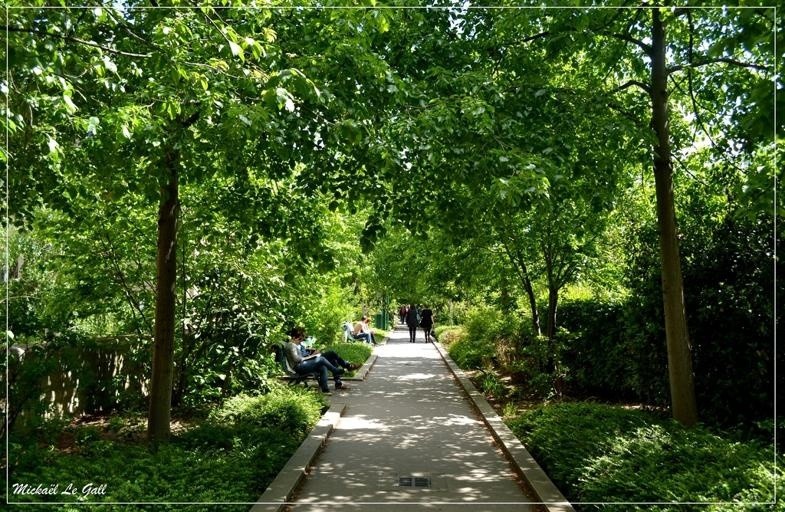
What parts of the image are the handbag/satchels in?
[416,314,420,321]
[432,330,435,337]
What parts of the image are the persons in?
[364,318,377,344]
[289,326,362,391]
[399,304,422,325]
[285,328,348,396]
[351,315,373,345]
[406,303,418,343]
[419,303,434,344]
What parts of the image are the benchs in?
[341,322,366,344]
[285,348,321,389]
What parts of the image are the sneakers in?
[321,391,332,396]
[348,363,360,371]
[336,384,349,389]
[334,368,347,375]
